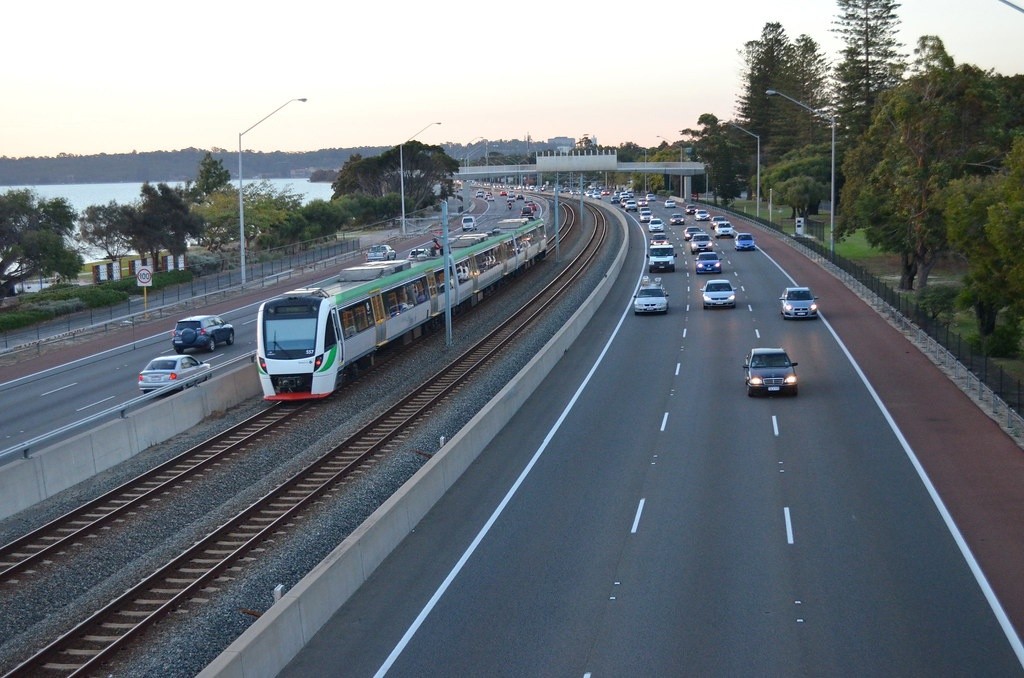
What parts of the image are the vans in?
[461,216,477,231]
[646,243,678,272]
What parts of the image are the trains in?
[253,215,547,402]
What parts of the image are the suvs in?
[172,314,235,354]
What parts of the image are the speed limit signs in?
[137,269,153,284]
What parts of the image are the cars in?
[743,347,799,396]
[734,232,755,251]
[689,233,713,254]
[650,233,671,246]
[647,218,665,232]
[665,199,677,208]
[685,204,697,215]
[365,245,396,262]
[709,215,736,239]
[407,248,429,264]
[468,180,657,223]
[694,210,710,221]
[699,279,738,308]
[779,287,819,319]
[683,226,705,240]
[694,252,723,274]
[669,214,684,226]
[138,354,212,394]
[633,276,670,315]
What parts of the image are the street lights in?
[454,135,484,209]
[237,97,307,286]
[765,89,835,261]
[716,122,760,219]
[398,121,442,237]
[655,135,683,199]
